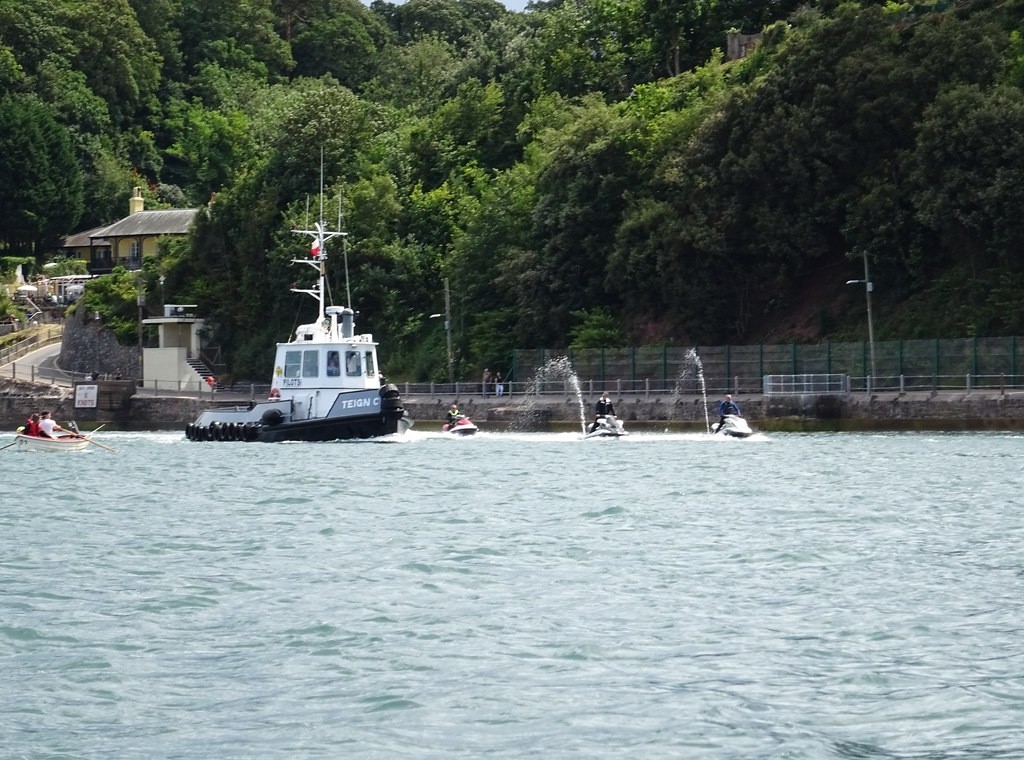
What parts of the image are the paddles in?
[61,428,115,451]
[0,442,16,451]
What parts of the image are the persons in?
[447,404,464,431]
[483,368,492,397]
[295,354,343,379]
[714,394,741,434]
[495,372,504,397]
[22,414,52,440]
[590,391,617,433]
[39,411,62,439]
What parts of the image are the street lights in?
[846,278,878,396]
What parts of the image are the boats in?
[585,412,625,438]
[442,415,478,436]
[14,425,89,451]
[710,411,753,437]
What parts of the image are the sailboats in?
[188,145,406,446]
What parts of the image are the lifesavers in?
[269,388,280,398]
